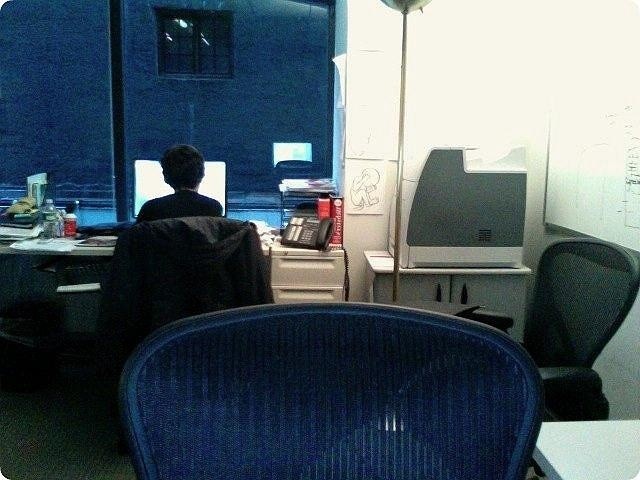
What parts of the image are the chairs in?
[117,302,546,480]
[468,236,640,476]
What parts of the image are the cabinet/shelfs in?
[364,250,531,344]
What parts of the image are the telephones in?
[281,214,334,251]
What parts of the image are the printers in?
[386,140,527,268]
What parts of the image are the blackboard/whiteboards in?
[542,91,640,257]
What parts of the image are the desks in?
[531,419,640,480]
[1,234,346,305]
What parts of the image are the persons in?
[132,143,223,226]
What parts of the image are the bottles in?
[42,198,77,239]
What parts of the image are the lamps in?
[381,0,432,304]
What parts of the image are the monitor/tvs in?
[133,159,227,220]
[274,142,314,170]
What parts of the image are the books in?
[1,173,49,242]
[279,178,343,244]
[75,239,116,247]
